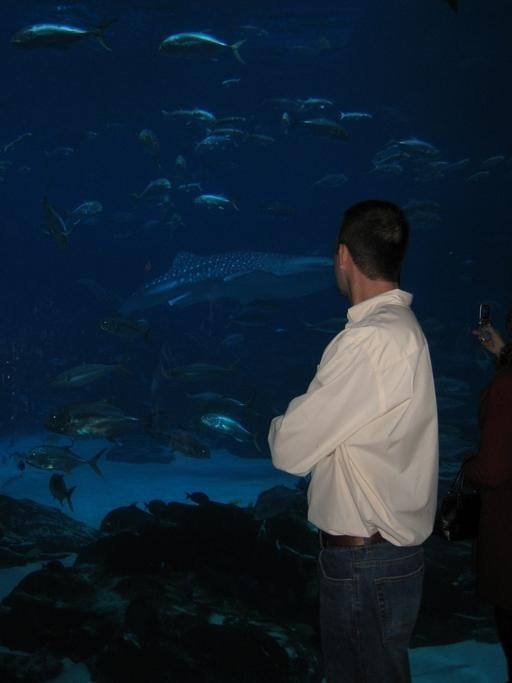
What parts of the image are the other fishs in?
[9,16,503,520]
[41,559,64,573]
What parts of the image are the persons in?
[468,316,512,682]
[264,197,444,682]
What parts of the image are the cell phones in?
[478,303,492,345]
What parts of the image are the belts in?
[319,530,387,548]
